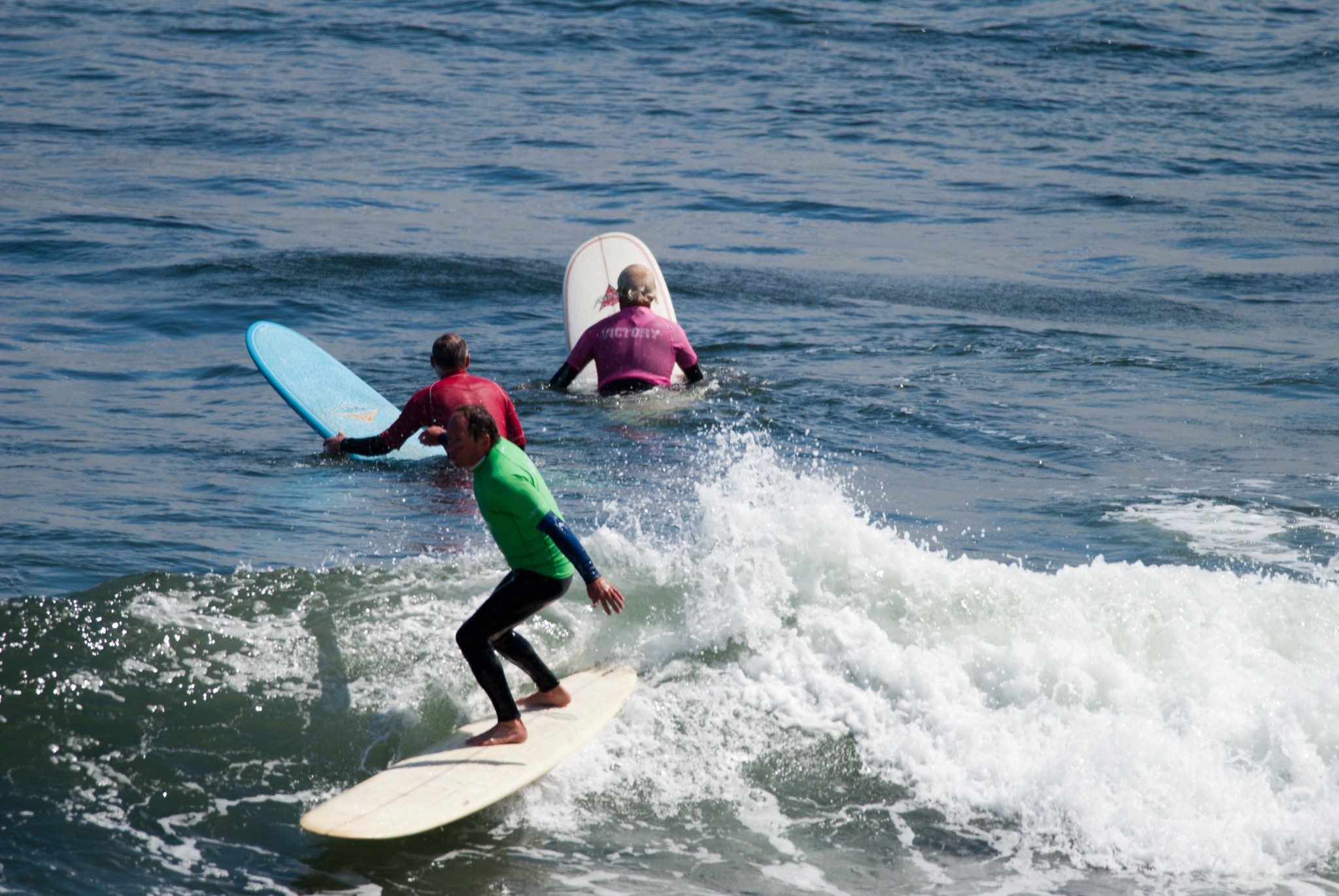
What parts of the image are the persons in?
[419,404,625,745]
[323,334,526,457]
[549,264,704,397]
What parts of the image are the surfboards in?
[295,663,638,840]
[245,317,452,470]
[561,230,680,410]
